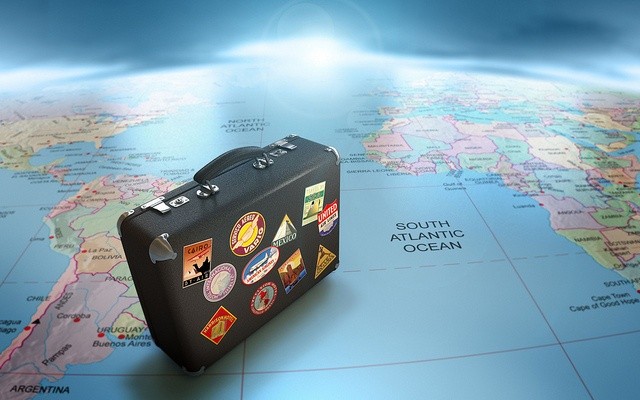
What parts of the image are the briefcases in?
[116,133,341,380]
